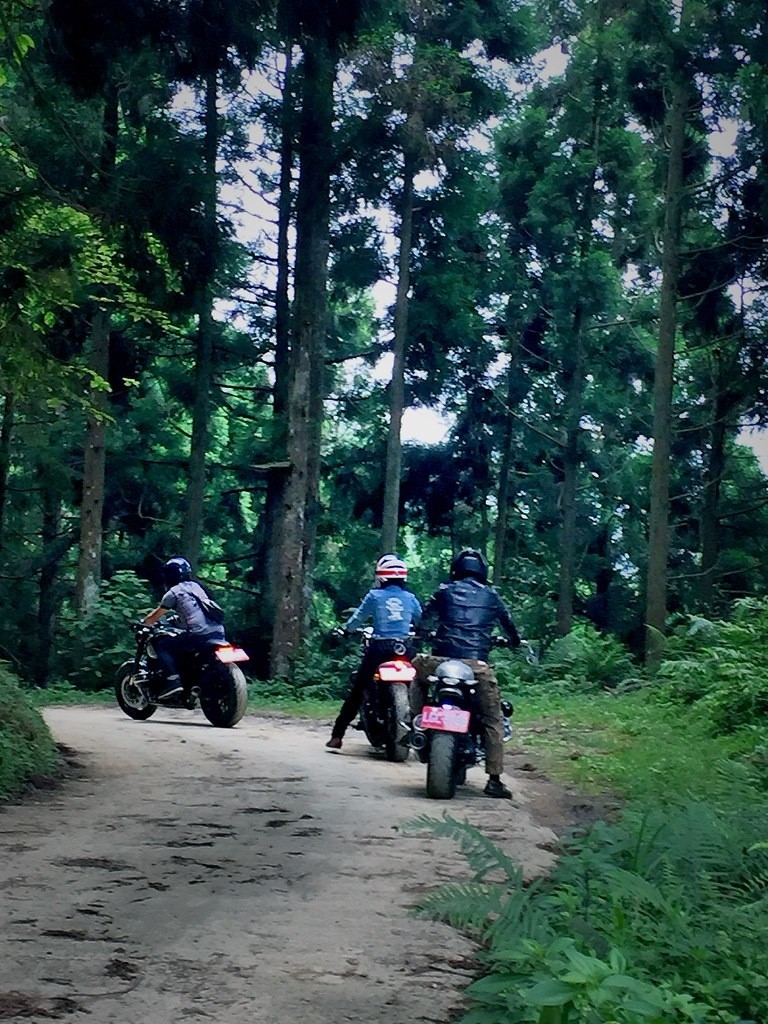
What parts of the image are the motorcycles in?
[329,624,423,762]
[114,613,250,728]
[394,632,535,799]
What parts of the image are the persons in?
[398,550,521,799]
[139,556,225,700]
[323,554,423,754]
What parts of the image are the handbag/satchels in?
[180,583,225,623]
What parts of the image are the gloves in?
[510,635,521,646]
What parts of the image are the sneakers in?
[326,738,342,749]
[483,780,513,799]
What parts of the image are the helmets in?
[164,558,192,581]
[450,549,489,581]
[374,553,408,588]
